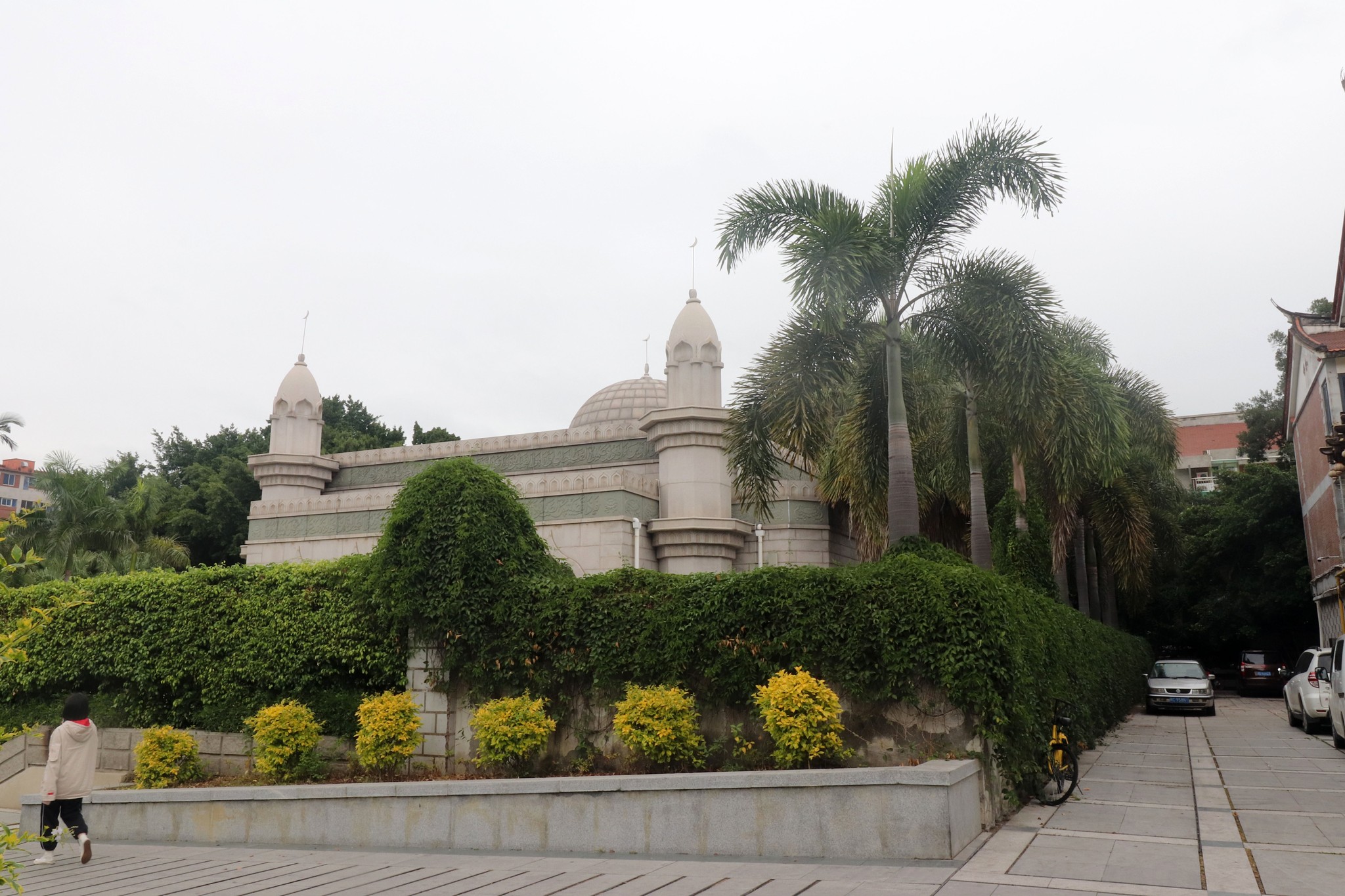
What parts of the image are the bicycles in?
[1031,696,1082,806]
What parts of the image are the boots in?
[33,850,55,864]
[78,834,92,864]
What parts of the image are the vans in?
[1314,634,1345,750]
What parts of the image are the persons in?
[33,692,98,865]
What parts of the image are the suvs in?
[1231,649,1288,697]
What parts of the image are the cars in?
[1279,646,1332,735]
[1141,660,1216,716]
